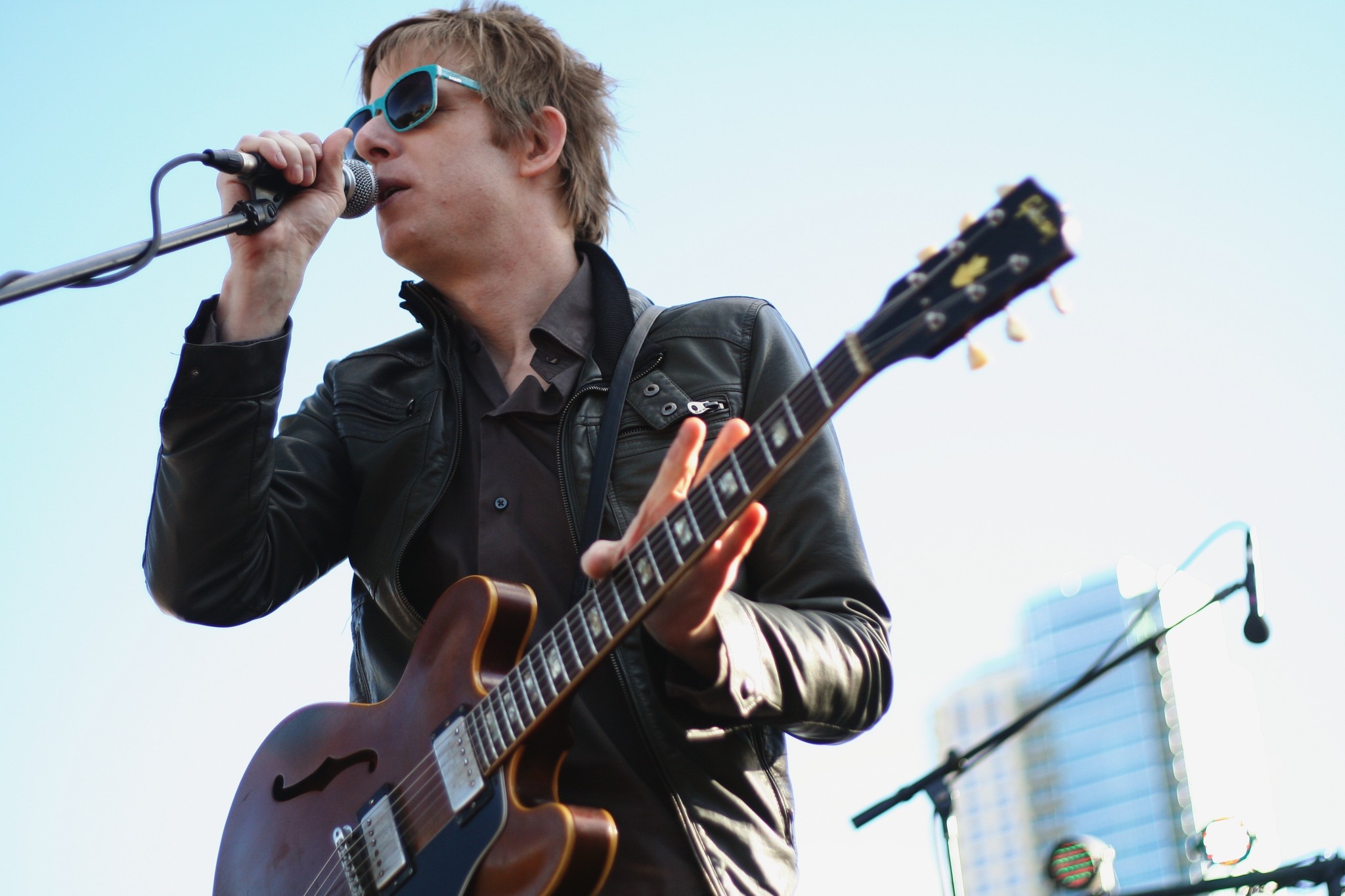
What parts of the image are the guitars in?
[212,178,1077,896]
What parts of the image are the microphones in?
[200,149,379,219]
[1243,531,1270,642]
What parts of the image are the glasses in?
[340,62,536,165]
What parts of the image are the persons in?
[139,0,887,893]
